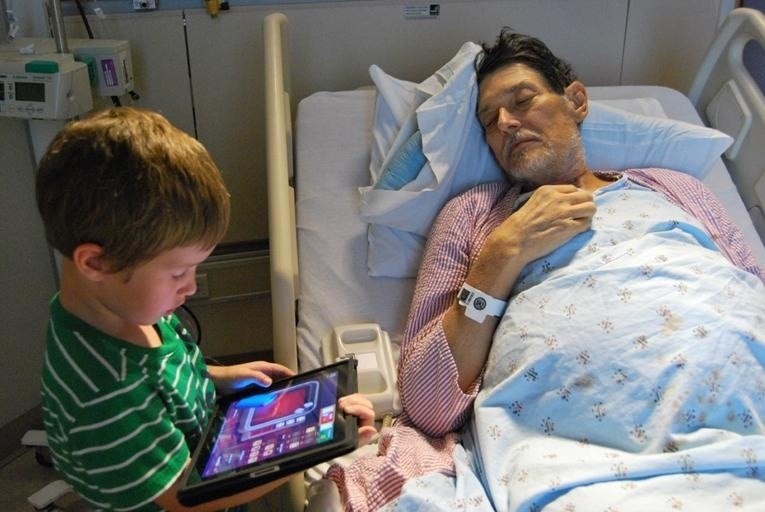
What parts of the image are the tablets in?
[174,358,359,508]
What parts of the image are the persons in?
[395,24,765,436]
[33,108,377,512]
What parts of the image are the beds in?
[260,7,764,512]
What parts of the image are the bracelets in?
[454,281,506,324]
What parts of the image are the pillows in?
[359,42,733,240]
[365,65,670,280]
